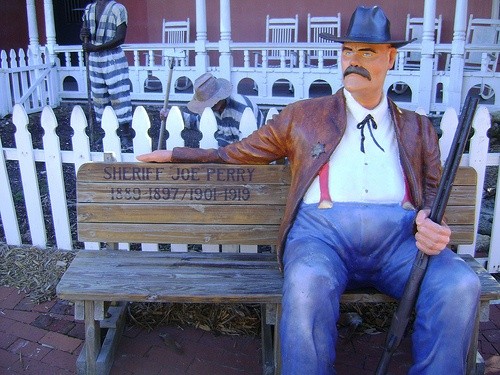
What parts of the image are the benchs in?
[56,161,500,375]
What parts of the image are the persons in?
[159,73,276,163]
[79,0,134,153]
[136,4,484,375]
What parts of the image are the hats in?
[187,73,233,114]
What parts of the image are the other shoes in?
[95,132,105,146]
[122,133,133,147]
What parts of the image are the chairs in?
[143,18,191,90]
[392,13,443,95]
[254,14,299,93]
[304,13,341,85]
[440,14,500,99]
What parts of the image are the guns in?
[376,94,479,375]
